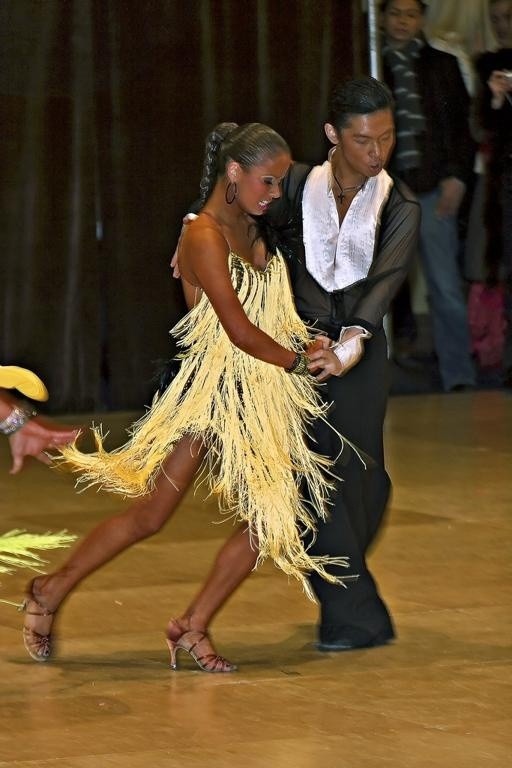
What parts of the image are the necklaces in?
[331,165,368,205]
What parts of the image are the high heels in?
[164,615,237,672]
[17,574,54,662]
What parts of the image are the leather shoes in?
[317,637,354,651]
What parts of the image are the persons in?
[169,76,421,651]
[18,119,338,674]
[369,0,478,394]
[0,356,85,484]
[468,0,512,388]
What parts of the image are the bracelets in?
[0,402,38,435]
[285,352,314,378]
